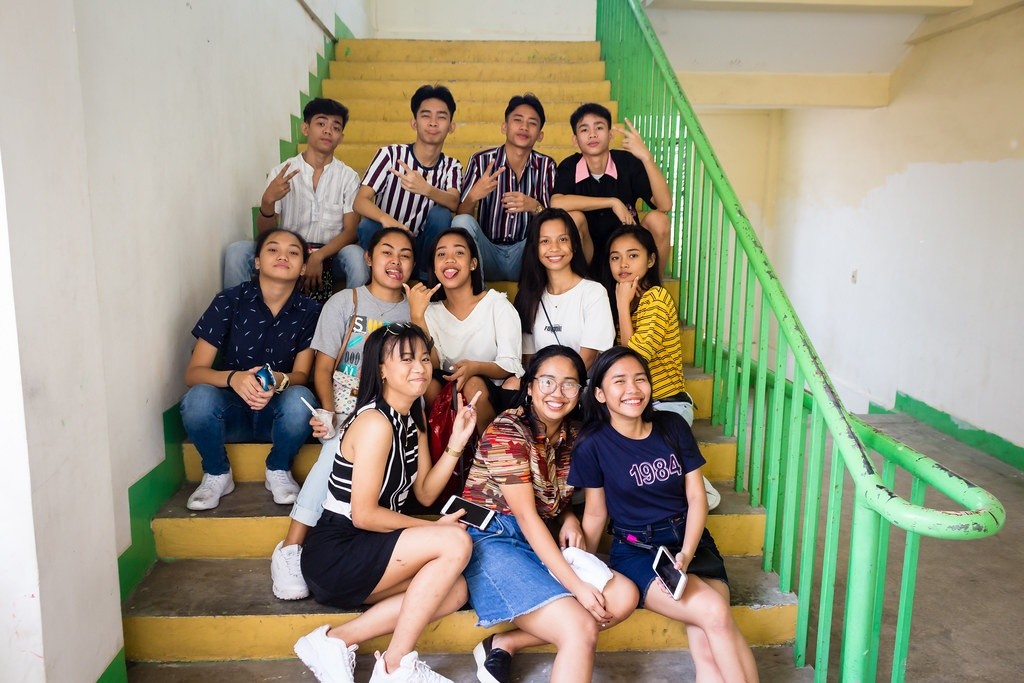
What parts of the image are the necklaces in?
[399,416,409,447]
[375,293,398,317]
[546,276,581,308]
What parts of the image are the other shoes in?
[570,486,585,505]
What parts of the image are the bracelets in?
[227,370,236,386]
[259,206,275,218]
[445,447,464,457]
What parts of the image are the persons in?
[180,228,327,510]
[294,323,482,683]
[270,226,413,600]
[566,346,760,683]
[400,227,526,502]
[223,78,704,291]
[513,208,616,369]
[461,344,640,683]
[601,224,721,511]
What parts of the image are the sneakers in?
[294,624,360,683]
[701,476,721,511]
[270,540,308,600]
[187,467,236,510]
[472,632,512,683]
[368,649,455,683]
[263,467,300,503]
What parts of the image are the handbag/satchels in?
[425,378,466,464]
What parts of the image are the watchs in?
[274,373,290,394]
[533,201,542,215]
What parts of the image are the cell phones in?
[441,495,495,531]
[652,546,688,601]
[255,362,276,391]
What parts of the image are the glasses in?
[532,375,582,399]
[384,320,416,339]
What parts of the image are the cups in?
[312,409,336,440]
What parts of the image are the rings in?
[467,403,476,410]
